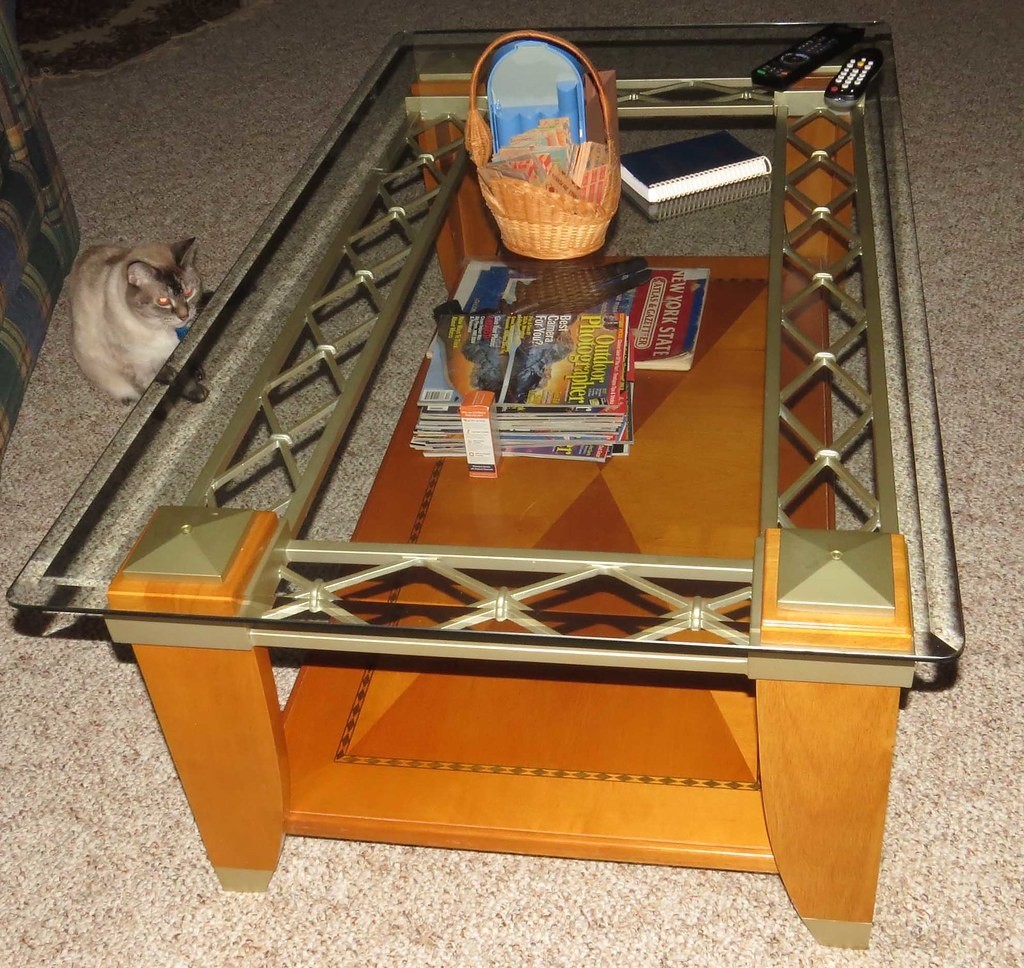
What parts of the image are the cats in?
[65,237,209,409]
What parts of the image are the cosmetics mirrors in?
[487,41,587,158]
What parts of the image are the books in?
[409,257,710,463]
[620,129,772,203]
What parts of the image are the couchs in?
[1,1,83,478]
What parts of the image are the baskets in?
[466,31,622,259]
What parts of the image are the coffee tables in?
[5,20,968,950]
